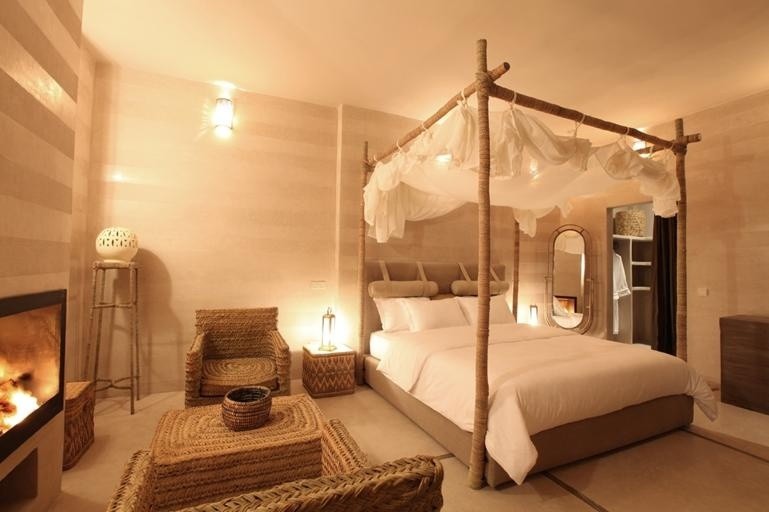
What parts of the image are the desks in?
[63,382,99,469]
[81,260,143,417]
[145,393,326,507]
[302,344,356,398]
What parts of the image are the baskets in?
[616,210,649,236]
[222,385,272,431]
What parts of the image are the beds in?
[360,37,719,490]
[553,313,583,330]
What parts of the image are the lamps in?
[528,304,540,328]
[213,95,236,143]
[320,307,341,352]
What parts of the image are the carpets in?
[111,418,367,512]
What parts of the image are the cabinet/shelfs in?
[720,316,768,419]
[611,232,653,342]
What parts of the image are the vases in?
[95,224,139,265]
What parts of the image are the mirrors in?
[546,223,594,337]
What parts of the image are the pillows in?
[553,295,568,316]
[407,300,469,332]
[375,295,435,332]
[453,295,516,329]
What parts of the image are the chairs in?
[167,455,450,512]
[181,304,291,406]
